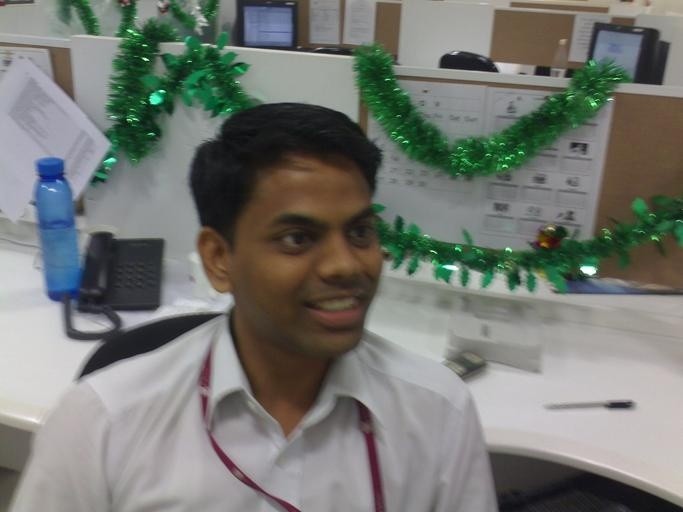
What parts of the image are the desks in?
[0,231,683,508]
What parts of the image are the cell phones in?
[441,350,487,379]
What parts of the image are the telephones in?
[78,232,164,313]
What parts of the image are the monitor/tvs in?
[232,0,298,49]
[588,22,659,85]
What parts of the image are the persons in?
[8,101,500,512]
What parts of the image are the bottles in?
[33,156,83,304]
[550,37,570,78]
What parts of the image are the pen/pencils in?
[546,401,634,410]
